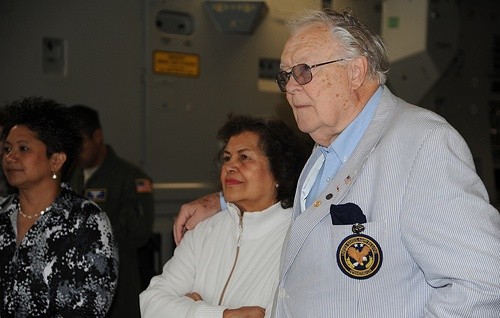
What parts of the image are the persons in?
[138,109,301,318]
[0,96,120,318]
[66,104,156,318]
[172,13,500,318]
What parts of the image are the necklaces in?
[17,199,54,219]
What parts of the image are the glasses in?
[275,59,344,92]
